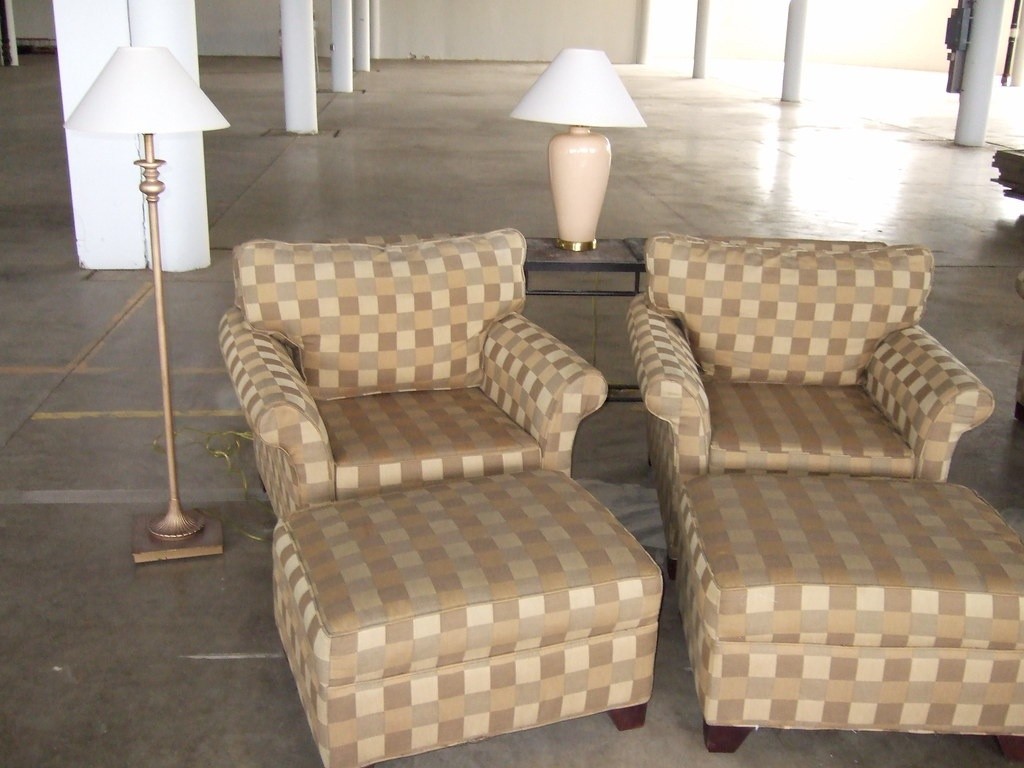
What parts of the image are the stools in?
[675,474,1024,760]
[271,469,663,768]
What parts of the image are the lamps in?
[63,47,230,563]
[511,49,647,252]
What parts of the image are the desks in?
[524,237,645,405]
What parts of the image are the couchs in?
[627,231,996,579]
[219,228,608,520]
